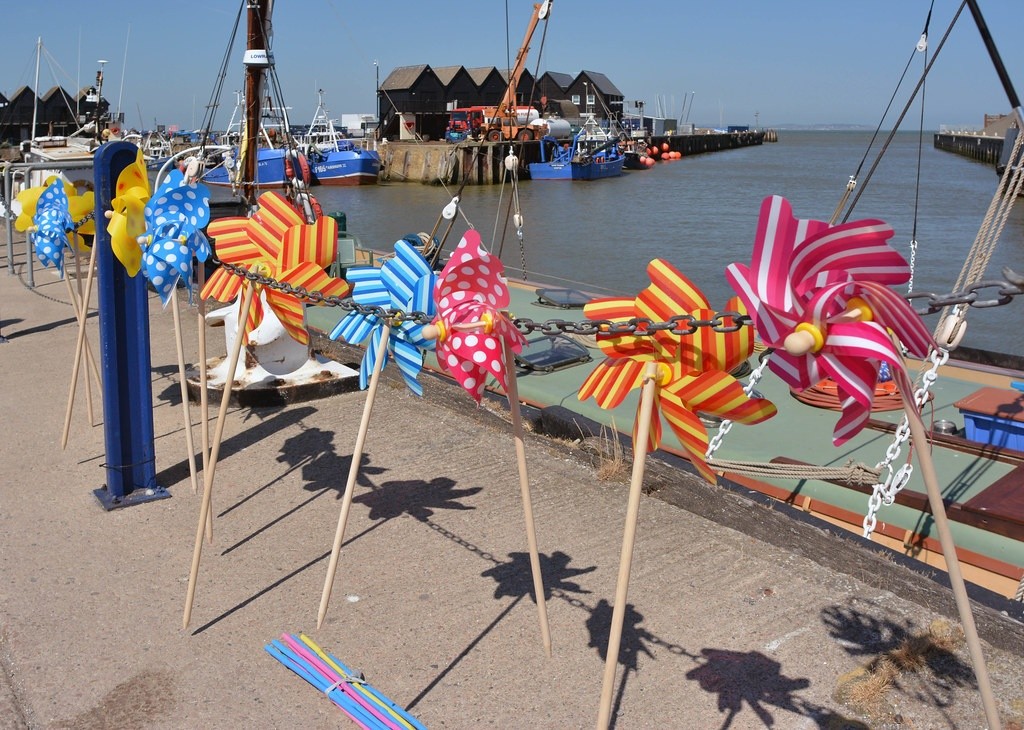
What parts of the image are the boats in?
[527,80,632,179]
[283,88,382,185]
[136,126,178,170]
[0,34,115,196]
[200,92,285,190]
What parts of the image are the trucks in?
[447,102,540,143]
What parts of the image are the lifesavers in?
[405,123,414,129]
[284,149,310,189]
[177,155,200,185]
[295,193,322,225]
